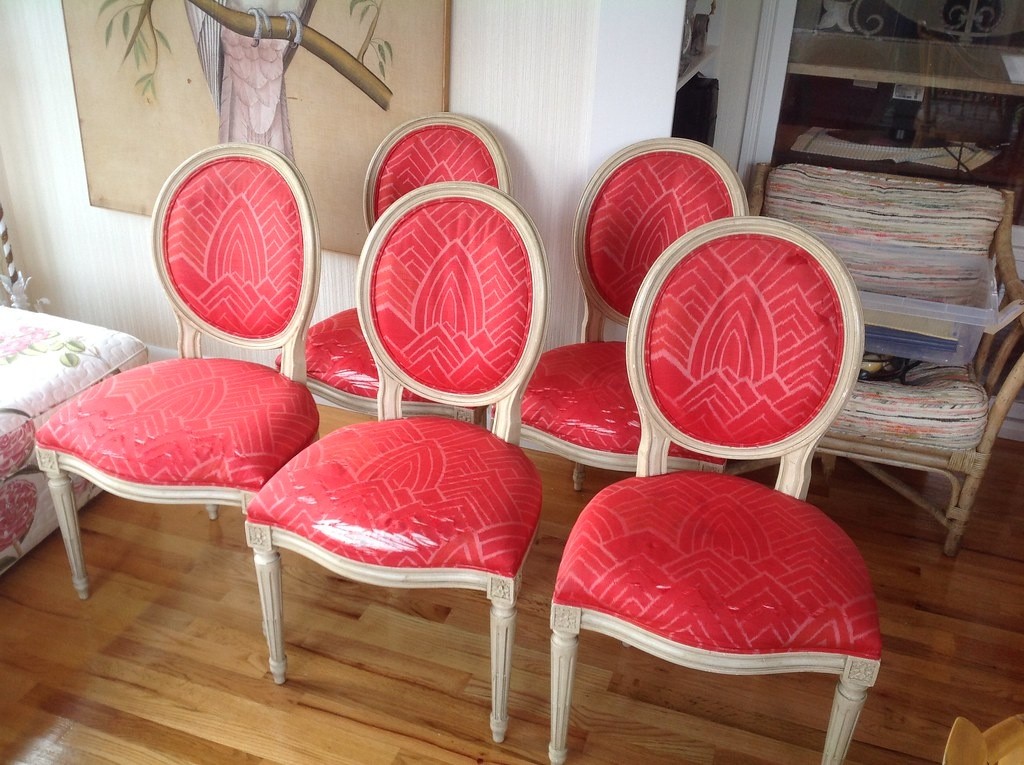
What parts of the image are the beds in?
[0,304,149,572]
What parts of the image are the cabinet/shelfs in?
[446,0,798,452]
[774,28,1024,442]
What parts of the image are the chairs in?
[34,142,322,603]
[549,214,881,765]
[245,181,543,744]
[726,162,1024,558]
[486,137,749,491]
[274,113,513,426]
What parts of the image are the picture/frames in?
[58,0,452,255]
